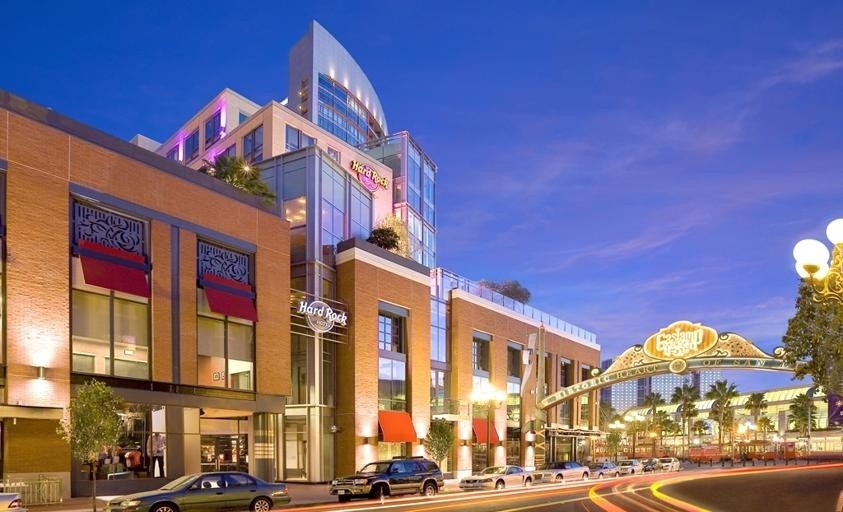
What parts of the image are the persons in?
[147,432,166,477]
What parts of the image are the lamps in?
[528,441,535,447]
[36,367,46,379]
[463,440,472,446]
[420,439,427,446]
[498,440,504,445]
[329,425,337,433]
[365,437,377,445]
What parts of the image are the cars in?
[106,471,291,511]
[533,461,590,483]
[459,465,535,490]
[590,456,681,480]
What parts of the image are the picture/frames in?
[213,372,220,380]
[221,371,225,380]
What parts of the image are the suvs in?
[329,456,445,503]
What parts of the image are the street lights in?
[468,383,509,467]
[609,411,645,461]
[740,421,757,452]
